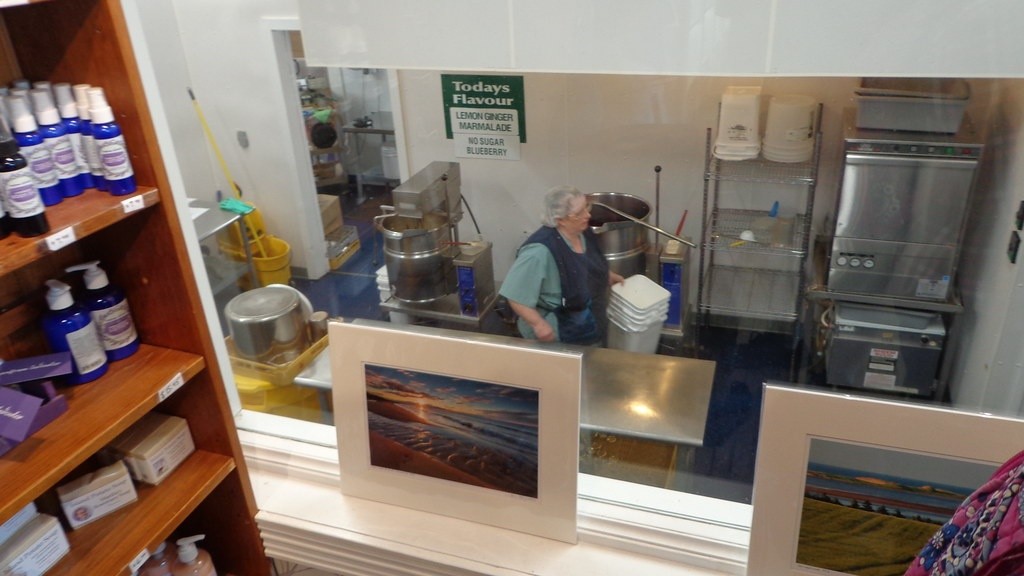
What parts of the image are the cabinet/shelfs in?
[0,0,282,576]
[694,102,825,384]
[186,196,260,338]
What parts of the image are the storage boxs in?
[223,317,344,386]
[325,225,361,271]
[318,194,343,236]
[854,79,977,132]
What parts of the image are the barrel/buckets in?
[380,213,457,305]
[226,287,304,365]
[249,234,290,285]
[711,86,762,162]
[586,193,651,275]
[763,95,817,161]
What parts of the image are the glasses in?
[566,204,592,221]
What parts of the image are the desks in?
[342,111,394,205]
[294,318,717,489]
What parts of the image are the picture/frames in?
[324,315,583,546]
[746,382,1024,576]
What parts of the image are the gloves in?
[217,197,255,215]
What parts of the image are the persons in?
[498,183,625,350]
[904,449,1024,576]
